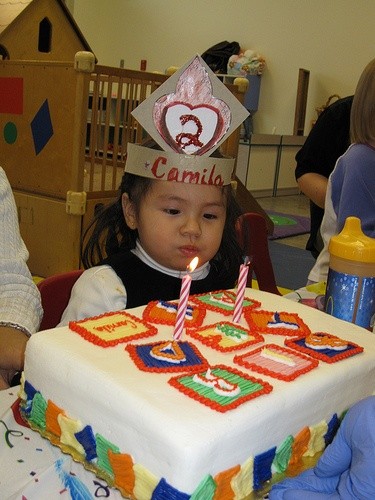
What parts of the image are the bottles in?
[323,216,375,330]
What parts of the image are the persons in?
[0,166,44,389]
[64,146,245,328]
[294,95,355,257]
[307,57,375,286]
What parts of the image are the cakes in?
[18,286,375,500]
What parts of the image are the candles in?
[233,256,250,322]
[174,256,199,341]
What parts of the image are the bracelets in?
[0,322,32,337]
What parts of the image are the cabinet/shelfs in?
[86,91,158,167]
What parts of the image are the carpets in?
[263,209,311,239]
[267,239,315,290]
